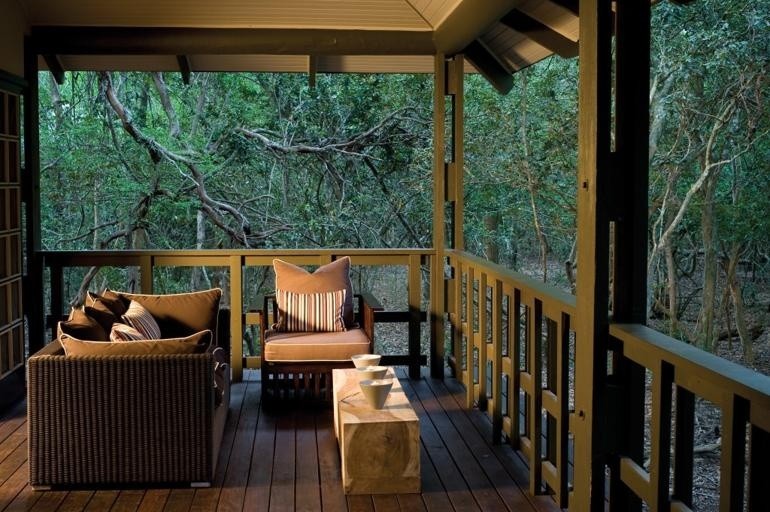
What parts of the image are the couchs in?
[26,307,230,491]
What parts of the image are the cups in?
[358,379,394,410]
[350,353,382,369]
[356,365,388,381]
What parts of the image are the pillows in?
[56,285,222,355]
[276,289,347,332]
[272,256,360,329]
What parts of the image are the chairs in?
[247,293,385,407]
